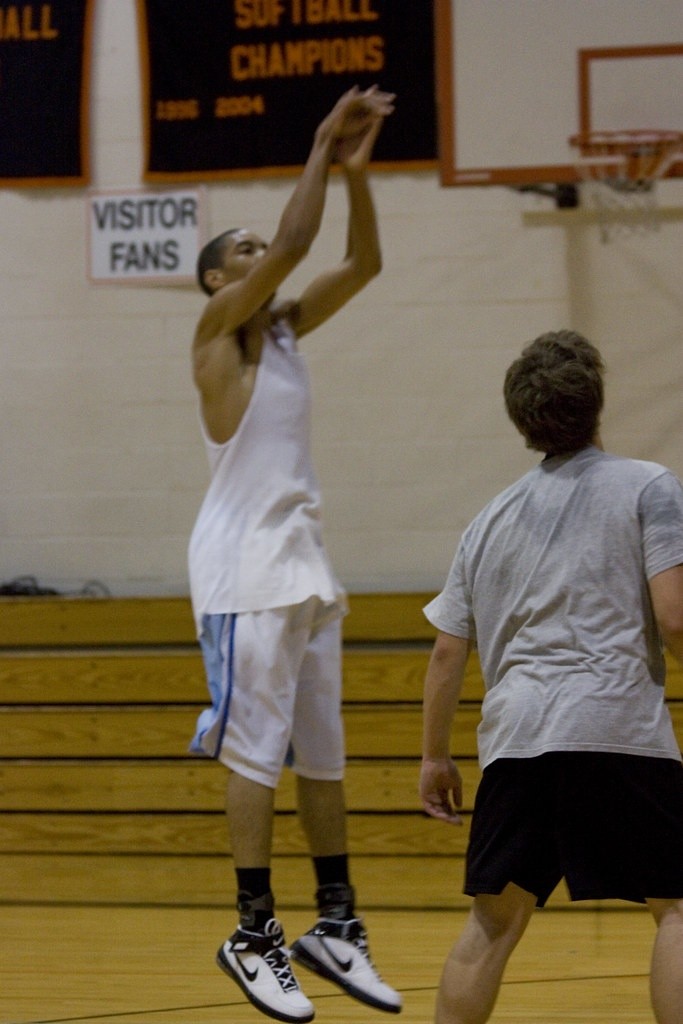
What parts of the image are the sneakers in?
[212,920,315,1024]
[290,919,404,1014]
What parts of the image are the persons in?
[188,84,406,1024]
[415,327,683,1024]
